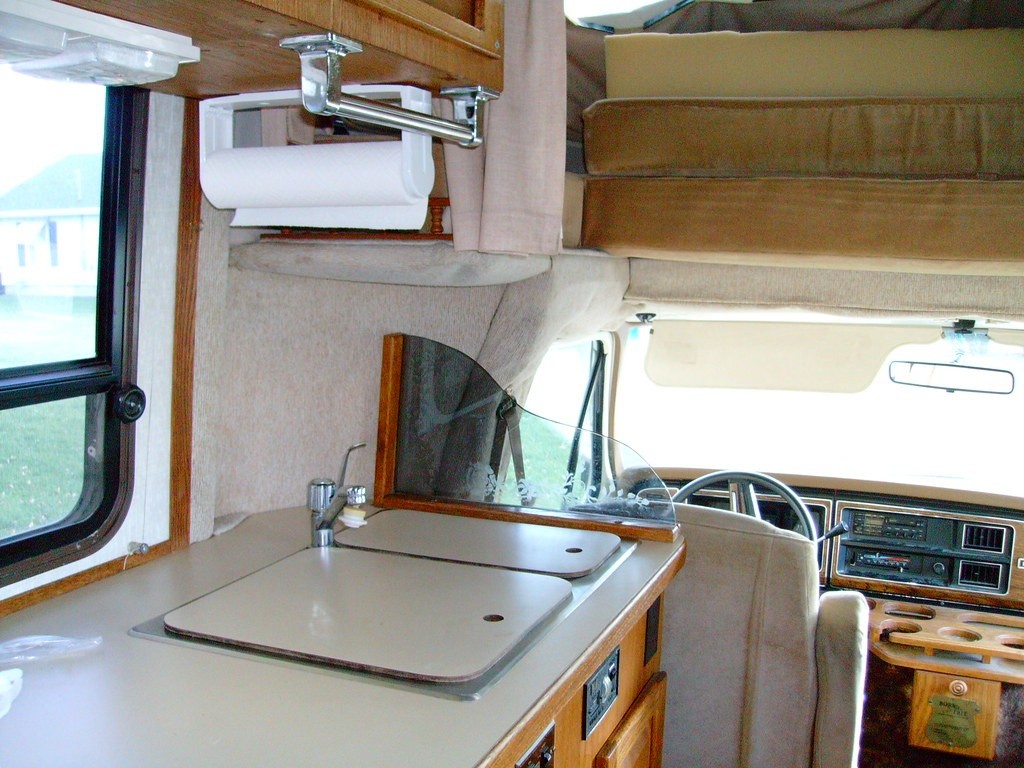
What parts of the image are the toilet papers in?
[200,141,428,230]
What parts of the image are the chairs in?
[572,500,869,768]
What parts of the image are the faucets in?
[337,442,367,487]
[306,478,366,548]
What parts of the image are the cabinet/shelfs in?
[51,0,506,99]
[513,589,667,768]
